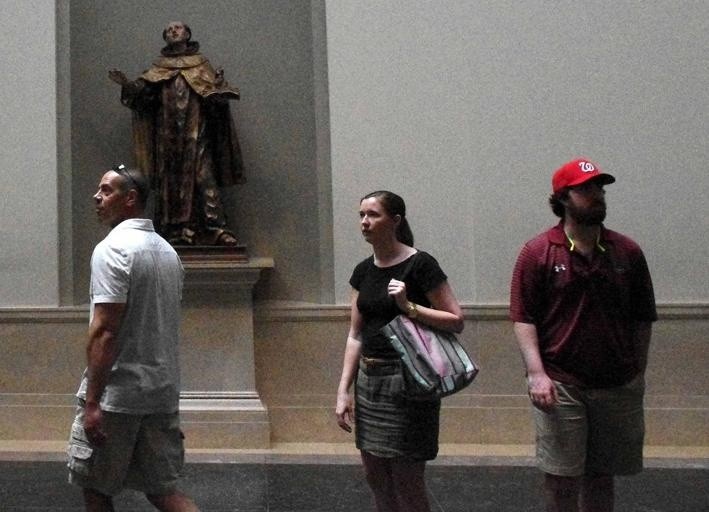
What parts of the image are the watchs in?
[408,302,418,318]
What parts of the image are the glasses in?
[112,162,141,194]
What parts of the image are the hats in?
[551,159,615,192]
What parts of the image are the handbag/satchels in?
[377,312,479,399]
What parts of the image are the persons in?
[67,170,200,511]
[108,21,243,245]
[335,190,464,510]
[510,159,658,512]
